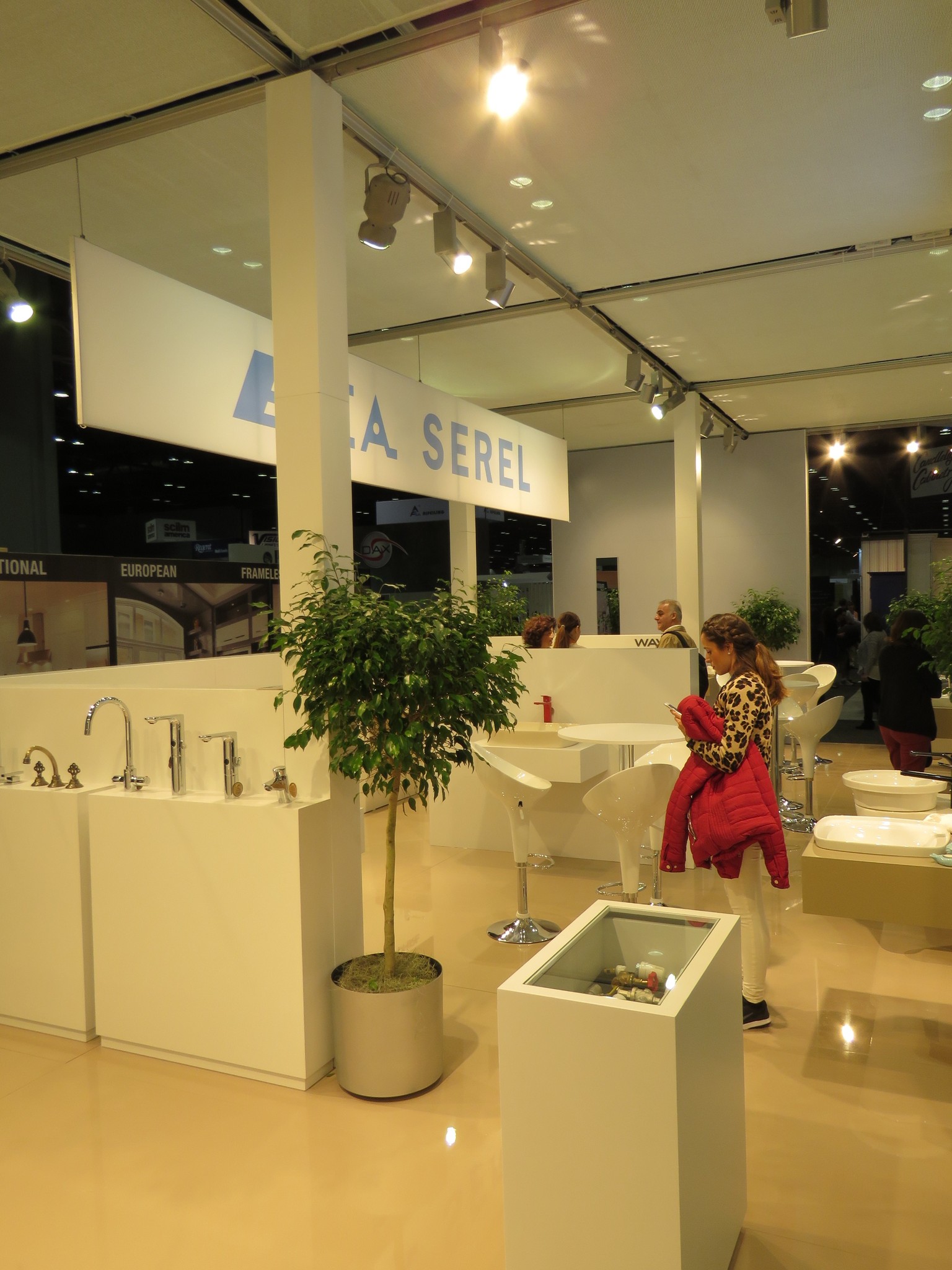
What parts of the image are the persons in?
[875,608,942,772]
[857,611,889,730]
[655,599,709,701]
[659,608,793,1030]
[521,611,587,649]
[811,598,861,670]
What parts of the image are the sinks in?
[813,813,951,858]
[487,722,581,748]
[842,769,949,813]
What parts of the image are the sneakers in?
[742,995,772,1030]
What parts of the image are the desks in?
[557,724,686,768]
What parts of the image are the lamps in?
[0,246,34,323]
[485,250,516,310]
[433,204,472,275]
[723,427,738,453]
[358,161,411,250]
[622,353,687,420]
[700,410,715,438]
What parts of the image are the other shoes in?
[857,719,874,730]
[831,683,838,688]
[849,665,859,670]
[839,679,854,686]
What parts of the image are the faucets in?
[263,765,298,804]
[143,714,188,796]
[83,695,150,792]
[899,751,952,809]
[23,746,66,788]
[199,730,244,799]
[534,693,553,722]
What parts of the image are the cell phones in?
[664,702,682,716]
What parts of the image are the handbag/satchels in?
[925,742,932,768]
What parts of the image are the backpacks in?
[661,631,709,700]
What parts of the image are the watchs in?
[685,733,692,742]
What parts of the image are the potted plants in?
[250,529,525,1099]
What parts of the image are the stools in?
[467,741,560,943]
[579,741,690,910]
[773,664,845,834]
